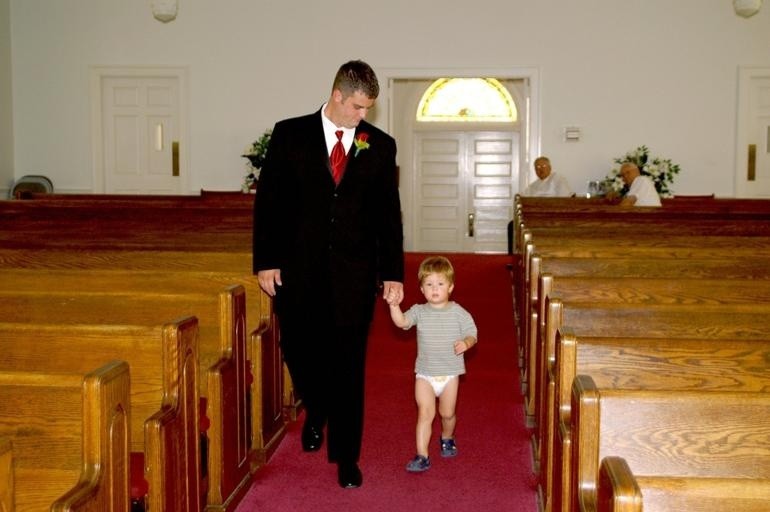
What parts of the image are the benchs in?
[2,192,303,512]
[509,191,770,511]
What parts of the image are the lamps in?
[150,1,178,22]
[733,1,762,18]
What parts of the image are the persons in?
[503,154,578,271]
[249,58,406,489]
[385,253,480,473]
[615,160,664,209]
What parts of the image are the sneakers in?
[408,454,431,472]
[440,437,458,458]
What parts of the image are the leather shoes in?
[337,459,363,490]
[301,412,326,452]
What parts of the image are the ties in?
[329,129,347,187]
